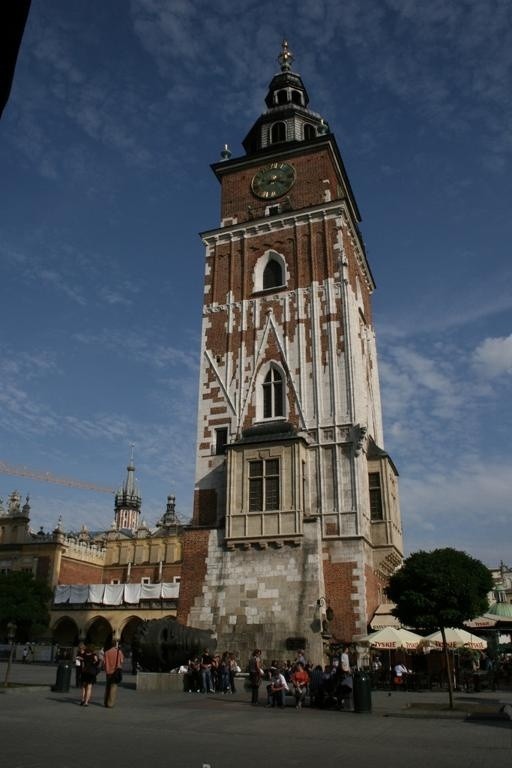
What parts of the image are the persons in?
[390,661,408,692]
[293,648,307,667]
[72,639,124,709]
[248,648,264,706]
[184,647,243,696]
[263,660,355,712]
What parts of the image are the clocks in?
[250,161,297,201]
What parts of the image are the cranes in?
[0,460,113,493]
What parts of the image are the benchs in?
[266,684,344,705]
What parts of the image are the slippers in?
[80,700,89,706]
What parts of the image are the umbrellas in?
[358,627,442,671]
[414,624,490,688]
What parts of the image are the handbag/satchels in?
[84,663,100,679]
[295,681,302,699]
[233,665,242,672]
[201,663,211,671]
[111,668,122,684]
[74,656,81,667]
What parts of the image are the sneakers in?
[196,689,201,693]
[203,688,233,695]
[104,702,115,708]
[189,689,192,693]
[264,703,286,710]
[296,702,303,712]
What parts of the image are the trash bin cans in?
[353,671,372,713]
[53,662,71,693]
